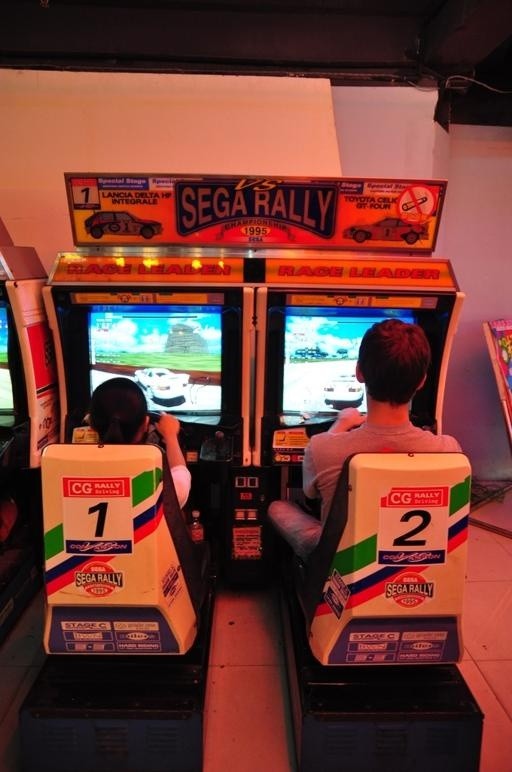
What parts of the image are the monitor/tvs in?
[0,303,16,415]
[87,304,223,416]
[282,306,418,414]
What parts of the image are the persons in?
[266,317,463,561]
[87,378,192,513]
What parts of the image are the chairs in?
[292,452,475,666]
[36,441,210,661]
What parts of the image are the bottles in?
[186,507,204,545]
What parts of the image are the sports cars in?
[134,367,190,401]
[324,372,363,405]
